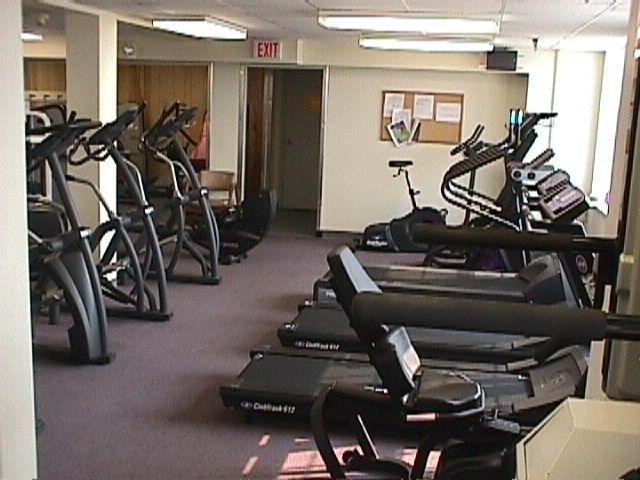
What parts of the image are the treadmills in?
[219,149,595,440]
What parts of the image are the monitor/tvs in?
[486,51,517,71]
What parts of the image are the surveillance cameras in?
[35,12,48,25]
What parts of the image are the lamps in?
[317,9,497,35]
[151,17,248,41]
[358,33,496,52]
[22,31,44,42]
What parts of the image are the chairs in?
[185,170,237,221]
